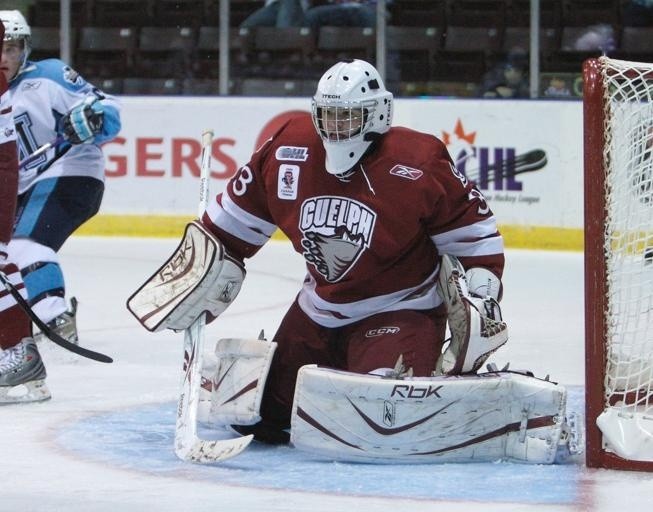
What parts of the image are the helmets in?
[1,9,33,83]
[311,53,393,184]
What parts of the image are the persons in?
[0,19,52,406]
[578,1,653,52]
[480,46,570,98]
[1,1,37,27]
[1,8,122,343]
[126,58,582,465]
[236,1,375,78]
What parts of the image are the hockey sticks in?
[0,272,116,363]
[173,128,254,464]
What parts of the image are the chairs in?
[30,1,652,101]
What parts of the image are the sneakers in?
[1,336,47,386]
[33,297,79,344]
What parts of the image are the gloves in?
[62,102,106,145]
